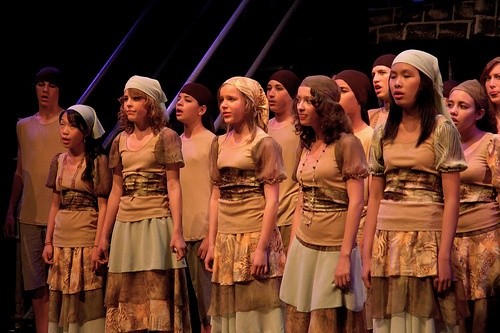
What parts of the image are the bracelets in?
[45,242,51,245]
[94,245,98,247]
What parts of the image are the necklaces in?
[58,155,84,205]
[299,143,327,226]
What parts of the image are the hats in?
[180,83,212,111]
[372,54,396,70]
[269,70,301,101]
[450,78,488,109]
[441,80,460,98]
[299,75,341,102]
[334,70,372,105]
[34,67,64,88]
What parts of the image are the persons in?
[203,76,287,333]
[173,82,218,333]
[278,74,370,333]
[263,70,301,251]
[4,67,65,333]
[95,75,193,333]
[360,50,469,333]
[445,79,500,333]
[480,56,500,134]
[332,70,376,333]
[41,104,112,333]
[366,54,396,129]
